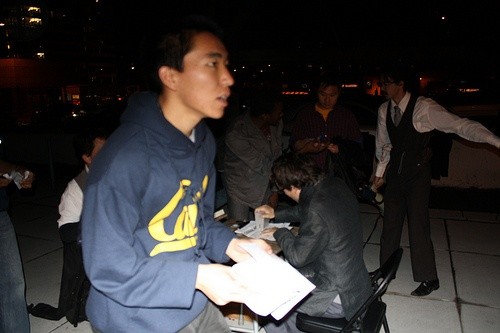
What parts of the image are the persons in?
[227,78,372,333]
[368,64,500,296]
[59,130,109,325]
[0,161,34,333]
[81,19,283,333]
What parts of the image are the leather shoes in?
[368,268,396,280]
[411,279,439,296]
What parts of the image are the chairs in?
[296,247,403,333]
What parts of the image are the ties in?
[394,105,401,127]
